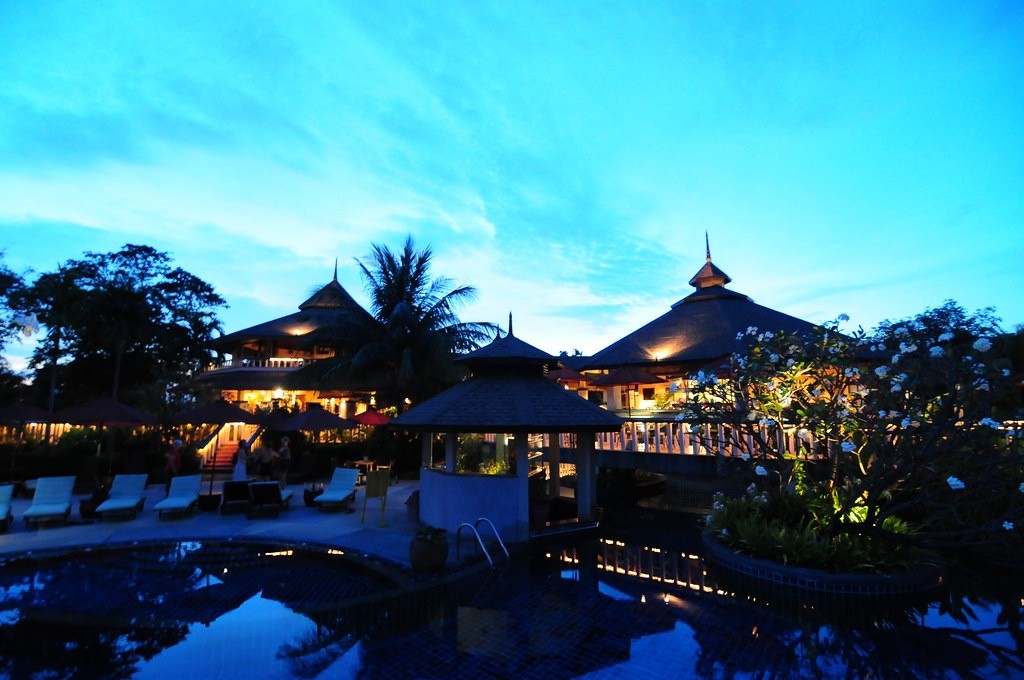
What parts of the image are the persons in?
[254,440,281,482]
[232,439,257,481]
[163,438,183,498]
[276,436,291,490]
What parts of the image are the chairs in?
[95,474,149,519]
[219,480,256,517]
[247,480,294,516]
[313,467,361,513]
[153,474,203,519]
[355,472,363,484]
[22,476,77,529]
[377,460,392,471]
[0,485,15,530]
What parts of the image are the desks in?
[353,460,375,484]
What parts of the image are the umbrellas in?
[0,397,396,516]
[547,368,592,383]
[587,364,671,418]
